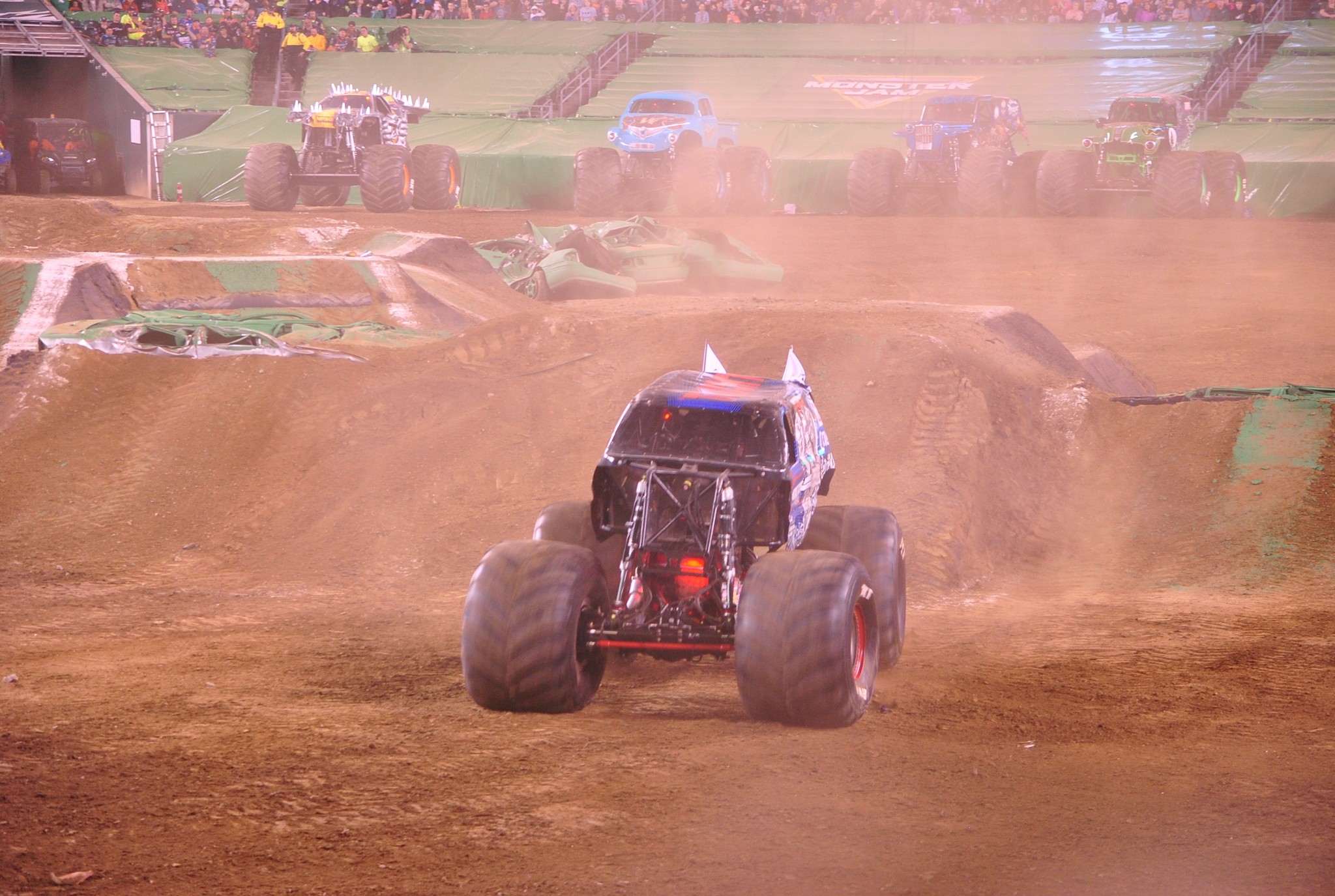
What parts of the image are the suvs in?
[1035,92,1247,224]
[243,81,463,216]
[459,341,912,729]
[843,94,1048,221]
[20,113,104,198]
[0,120,17,196]
[569,92,774,219]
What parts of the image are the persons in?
[65,0,1335,54]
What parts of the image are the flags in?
[781,349,806,385]
[704,344,727,374]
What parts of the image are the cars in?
[465,211,789,301]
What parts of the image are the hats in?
[224,8,231,12]
[192,18,200,23]
[268,3,276,10]
[339,27,346,32]
[100,16,107,23]
[146,26,153,31]
[178,22,186,28]
[128,6,134,11]
[289,22,296,27]
